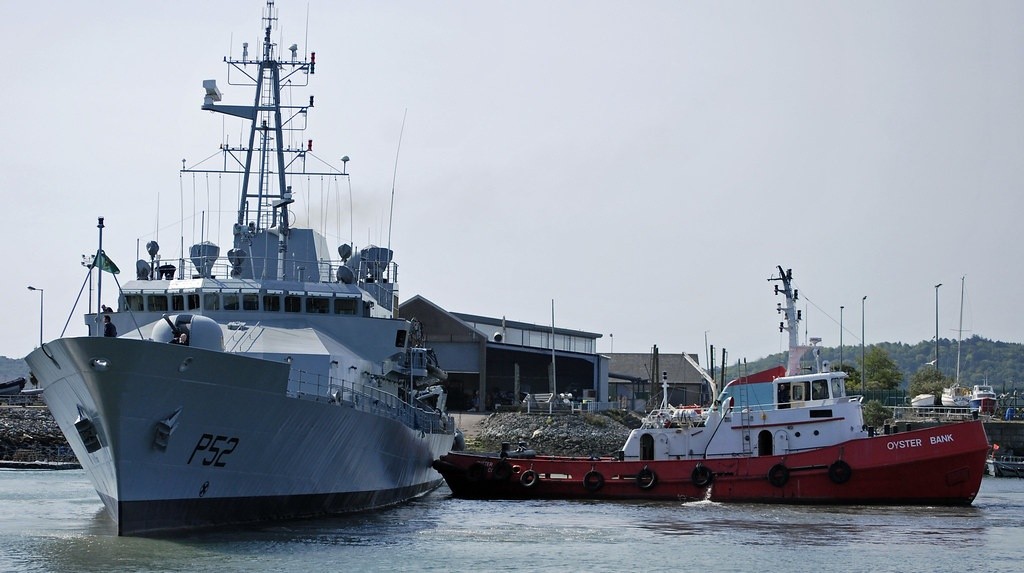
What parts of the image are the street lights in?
[27,285,43,344]
[935,283,943,370]
[862,295,867,394]
[839,305,844,372]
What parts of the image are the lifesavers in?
[827,458,854,486]
[519,469,540,489]
[689,465,714,489]
[766,463,791,489]
[634,468,658,491]
[493,459,514,482]
[582,469,606,494]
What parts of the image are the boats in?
[24,0,462,537]
[432,370,992,505]
[0,376,28,395]
[911,383,998,409]
[986,454,1024,479]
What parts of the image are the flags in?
[90,250,120,274]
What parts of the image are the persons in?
[169,333,187,346]
[778,385,789,409]
[102,315,117,337]
[155,265,176,280]
